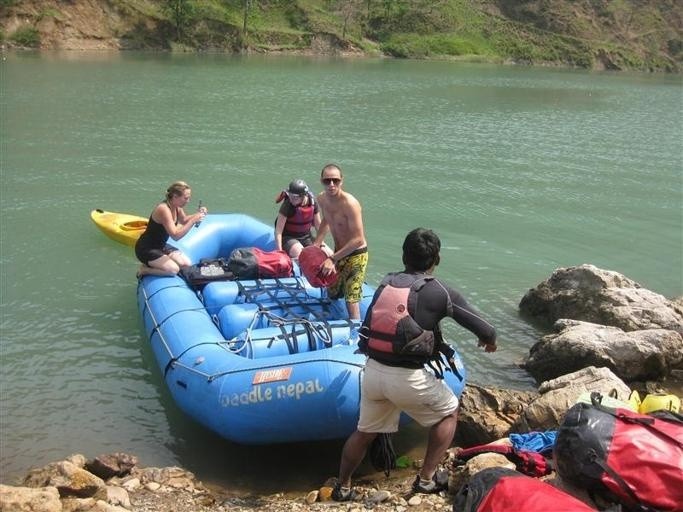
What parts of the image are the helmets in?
[287,179,308,196]
[601,387,681,414]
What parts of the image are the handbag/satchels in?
[552,400,683,512]
[187,258,232,284]
[451,467,600,512]
[228,246,293,279]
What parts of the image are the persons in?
[273,179,330,263]
[132,181,209,282]
[300,164,368,321]
[330,225,499,502]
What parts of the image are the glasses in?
[322,177,341,187]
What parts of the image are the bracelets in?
[329,254,338,264]
[190,217,194,225]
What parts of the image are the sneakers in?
[414,476,445,493]
[331,485,362,503]
